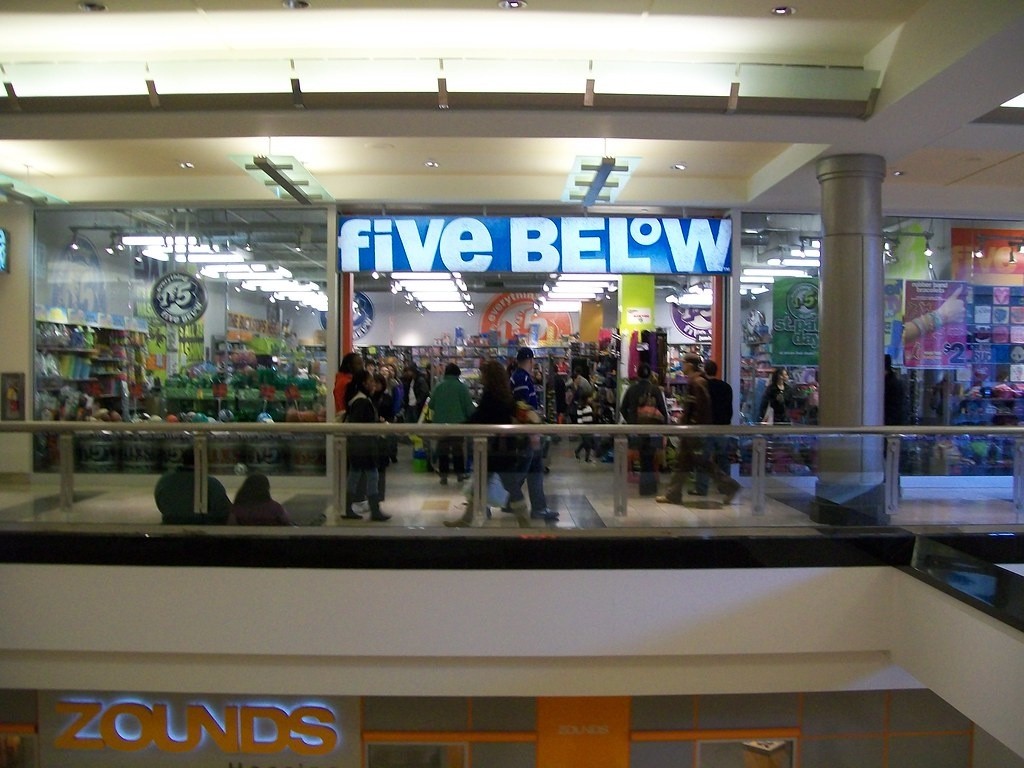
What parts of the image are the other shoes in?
[486,506,492,519]
[687,489,708,496]
[368,512,391,521]
[341,511,363,519]
[501,501,513,513]
[440,473,470,484]
[443,519,471,527]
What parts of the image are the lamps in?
[923,238,935,258]
[1008,241,1018,265]
[884,233,904,251]
[69,218,844,316]
[975,238,985,259]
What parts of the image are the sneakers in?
[656,495,683,503]
[723,481,742,503]
[530,508,560,519]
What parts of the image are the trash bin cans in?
[411,447,428,474]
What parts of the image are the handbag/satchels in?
[763,407,774,427]
[637,407,664,436]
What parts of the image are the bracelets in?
[913,312,944,335]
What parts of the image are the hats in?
[404,362,422,375]
[683,353,702,364]
[516,347,533,361]
[445,363,461,376]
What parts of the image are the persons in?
[762,368,797,425]
[155,448,292,525]
[902,283,966,341]
[884,354,904,426]
[333,347,743,518]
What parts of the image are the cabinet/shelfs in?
[35,316,147,399]
[370,342,572,390]
